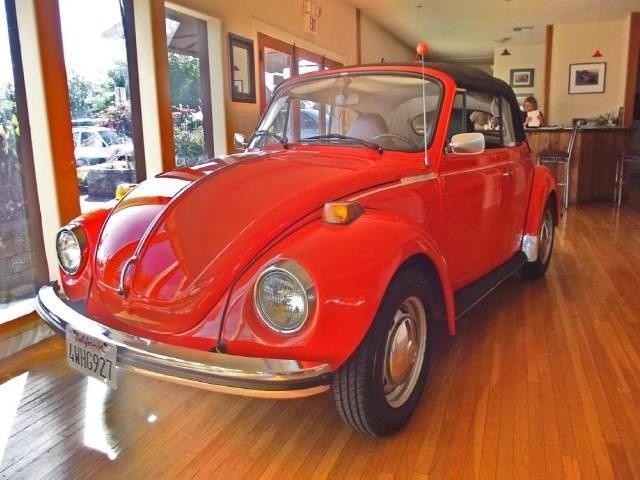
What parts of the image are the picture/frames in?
[570,62,606,94]
[234,79,243,93]
[510,68,535,112]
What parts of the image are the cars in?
[34,61,559,439]
[273,109,329,139]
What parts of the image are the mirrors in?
[228,31,257,103]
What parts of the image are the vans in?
[72,126,133,166]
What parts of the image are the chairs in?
[346,114,397,149]
[535,124,580,211]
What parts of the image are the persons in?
[520,97,545,128]
